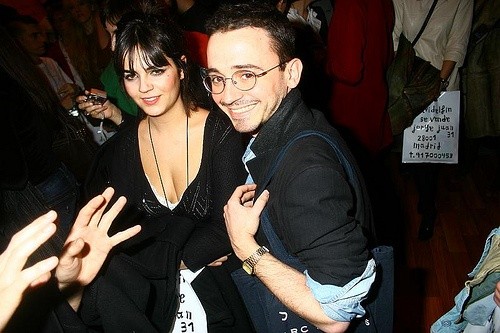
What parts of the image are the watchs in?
[241,245,269,275]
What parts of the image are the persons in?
[0,185,142,333]
[325,0,391,154]
[392,0,474,239]
[202,6,378,333]
[0,1,331,145]
[100,16,245,333]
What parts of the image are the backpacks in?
[386,31,442,133]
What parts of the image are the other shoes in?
[419,207,437,239]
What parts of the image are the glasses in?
[200,58,293,94]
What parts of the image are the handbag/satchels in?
[229,130,394,333]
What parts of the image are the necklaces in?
[148,115,190,217]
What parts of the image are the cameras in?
[85,88,107,107]
